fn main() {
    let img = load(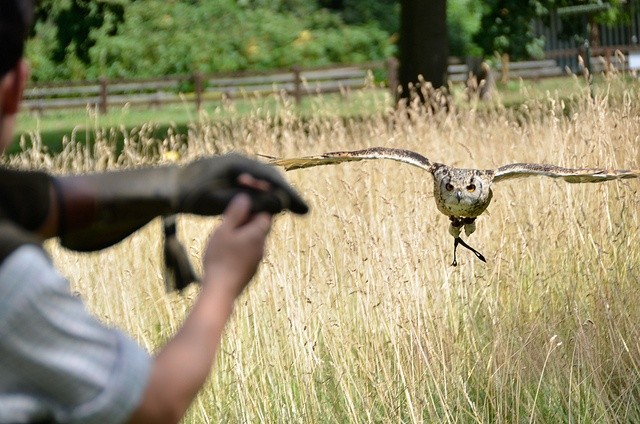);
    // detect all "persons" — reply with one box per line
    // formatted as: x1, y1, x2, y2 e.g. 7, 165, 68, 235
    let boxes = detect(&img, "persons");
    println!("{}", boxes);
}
0, 0, 309, 424
